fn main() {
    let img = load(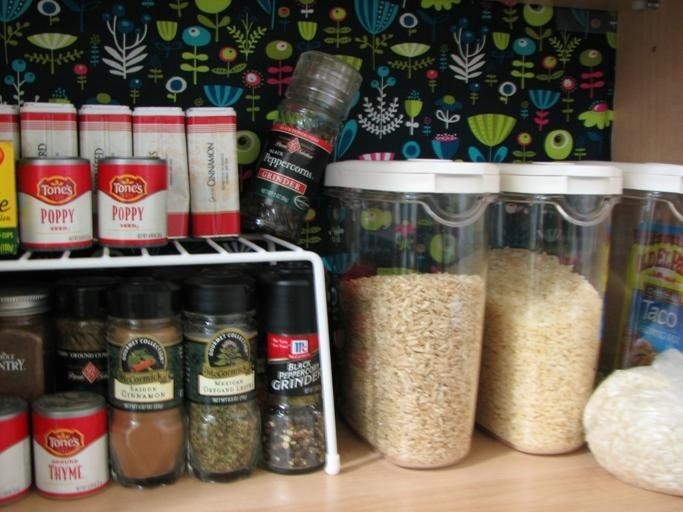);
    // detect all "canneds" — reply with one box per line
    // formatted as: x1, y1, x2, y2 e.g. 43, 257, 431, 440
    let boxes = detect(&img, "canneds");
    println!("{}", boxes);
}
1, 262, 326, 508
0, 102, 242, 252
